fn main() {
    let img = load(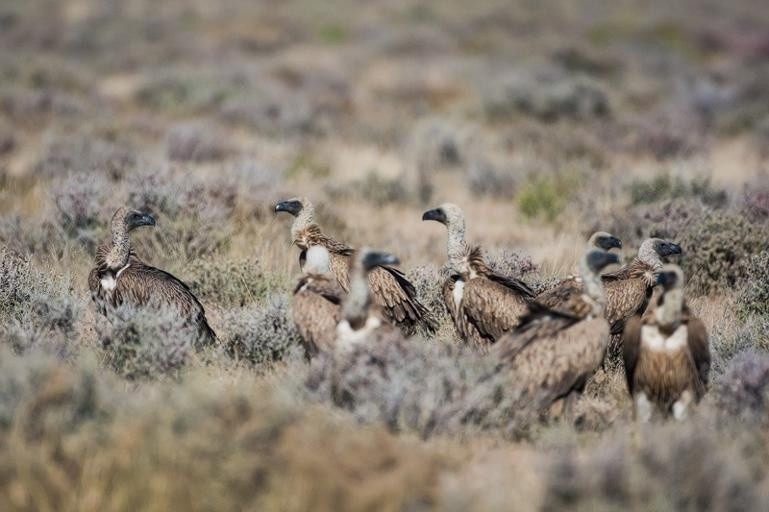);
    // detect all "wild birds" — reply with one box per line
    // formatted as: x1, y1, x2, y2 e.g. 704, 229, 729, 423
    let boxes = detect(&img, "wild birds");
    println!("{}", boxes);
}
85, 204, 222, 349
270, 195, 711, 426
